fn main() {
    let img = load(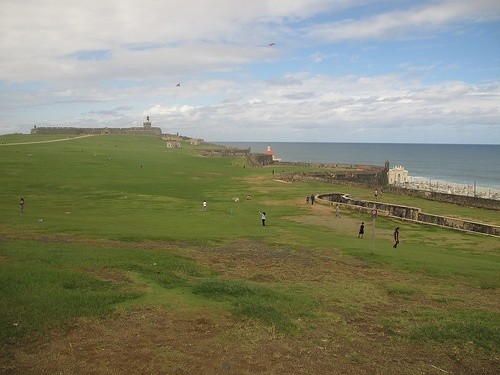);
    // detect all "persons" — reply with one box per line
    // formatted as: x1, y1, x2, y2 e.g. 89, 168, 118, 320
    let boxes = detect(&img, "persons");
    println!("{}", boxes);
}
306, 196, 309, 204
357, 222, 364, 239
393, 227, 399, 248
19, 198, 24, 215
201, 201, 208, 211
311, 194, 315, 205
335, 204, 340, 218
259, 211, 266, 226
